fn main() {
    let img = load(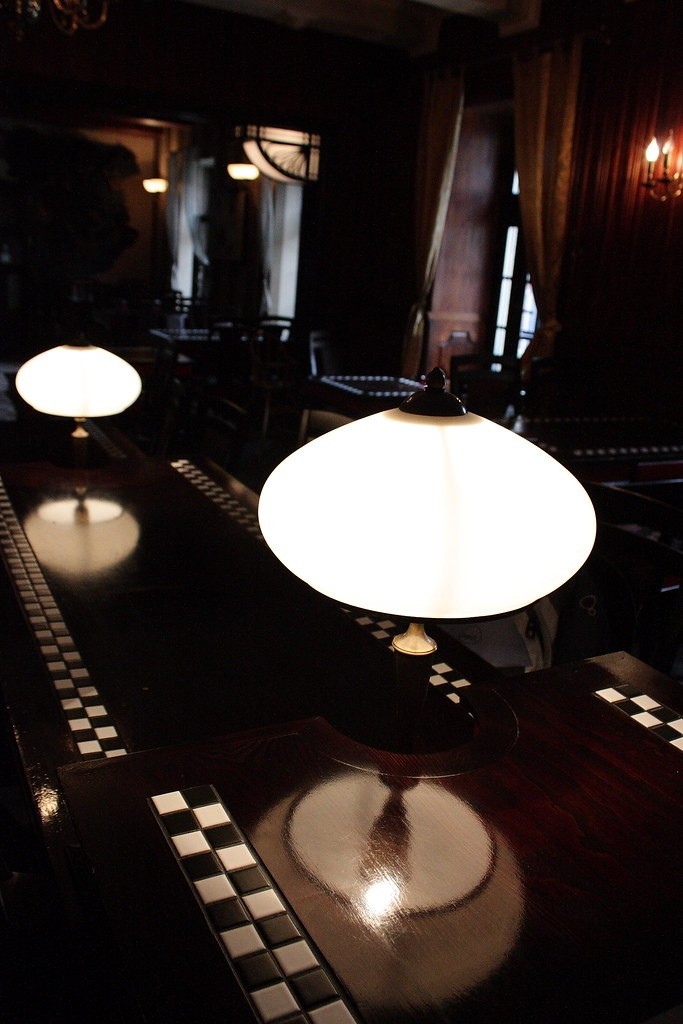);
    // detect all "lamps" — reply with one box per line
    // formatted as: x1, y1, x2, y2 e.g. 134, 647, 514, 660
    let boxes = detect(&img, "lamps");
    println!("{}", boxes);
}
236, 125, 322, 186
640, 129, 683, 205
16, 331, 142, 443
248, 772, 527, 1010
256, 357, 597, 785
24, 483, 142, 581
